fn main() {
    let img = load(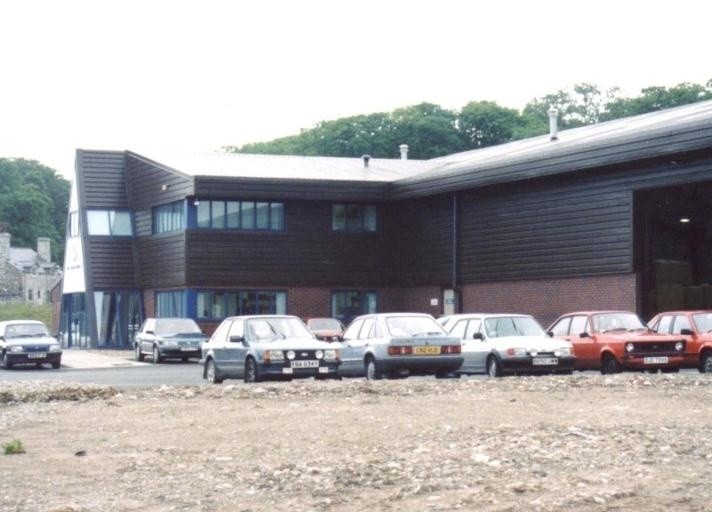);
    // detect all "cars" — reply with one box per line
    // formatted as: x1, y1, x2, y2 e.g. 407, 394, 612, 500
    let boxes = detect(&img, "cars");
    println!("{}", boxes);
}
441, 313, 574, 379
646, 310, 712, 374
133, 316, 209, 364
336, 312, 465, 380
306, 317, 346, 343
0, 319, 63, 369
545, 310, 687, 375
199, 314, 343, 383
